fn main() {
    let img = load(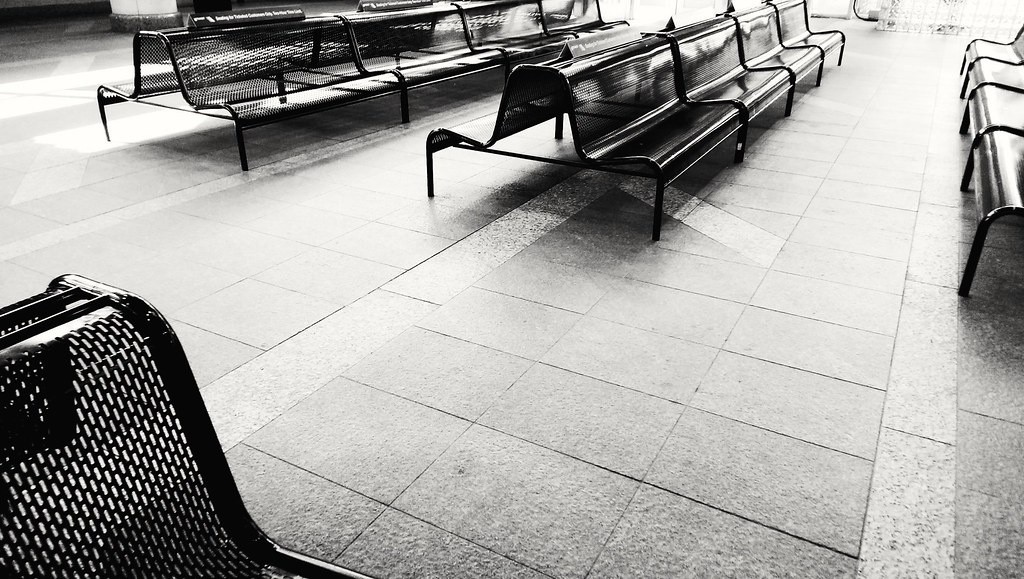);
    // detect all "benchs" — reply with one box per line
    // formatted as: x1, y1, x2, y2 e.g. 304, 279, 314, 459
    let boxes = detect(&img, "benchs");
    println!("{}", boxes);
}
96, 0, 848, 247
958, 24, 1024, 297
0, 273, 380, 579
